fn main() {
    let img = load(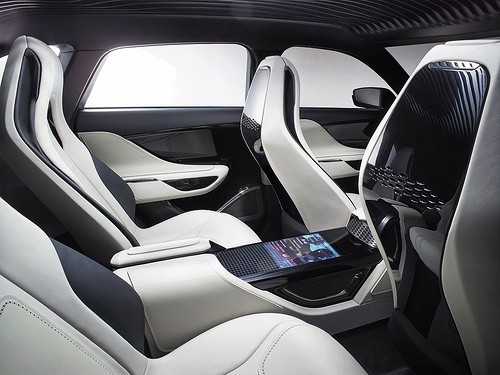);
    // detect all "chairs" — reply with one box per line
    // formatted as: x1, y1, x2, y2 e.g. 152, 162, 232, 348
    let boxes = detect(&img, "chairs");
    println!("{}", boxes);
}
0, 197, 370, 375
358, 39, 500, 375
0, 35, 262, 268
240, 55, 365, 237
